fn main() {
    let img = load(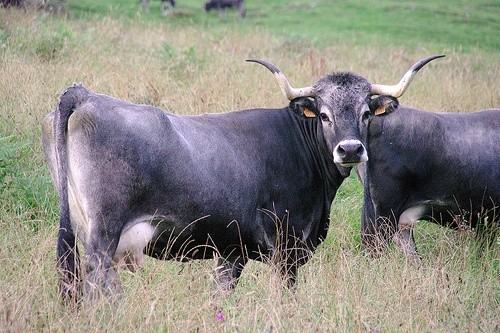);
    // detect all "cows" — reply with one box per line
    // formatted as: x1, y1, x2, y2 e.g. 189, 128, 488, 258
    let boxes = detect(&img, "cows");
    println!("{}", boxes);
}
355, 106, 500, 273
41, 54, 448, 308
